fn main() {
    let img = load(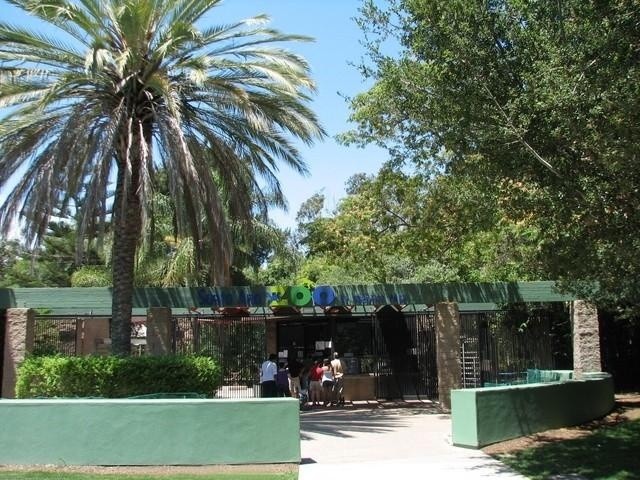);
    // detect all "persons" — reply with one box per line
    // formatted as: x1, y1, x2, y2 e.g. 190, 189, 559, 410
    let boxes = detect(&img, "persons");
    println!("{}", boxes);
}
330, 352, 347, 407
261, 353, 278, 398
284, 354, 304, 398
321, 358, 334, 407
309, 360, 323, 408
279, 362, 284, 372
